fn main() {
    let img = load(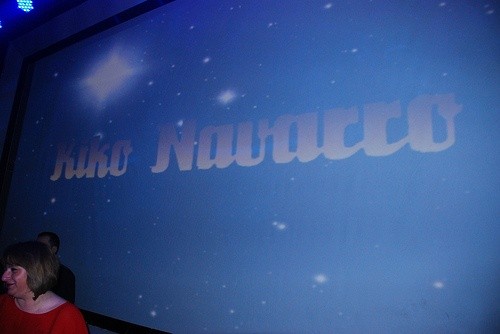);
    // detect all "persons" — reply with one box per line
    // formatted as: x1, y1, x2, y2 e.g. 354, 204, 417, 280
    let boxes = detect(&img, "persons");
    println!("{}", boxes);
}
0, 240, 89, 334
35, 232, 77, 305
